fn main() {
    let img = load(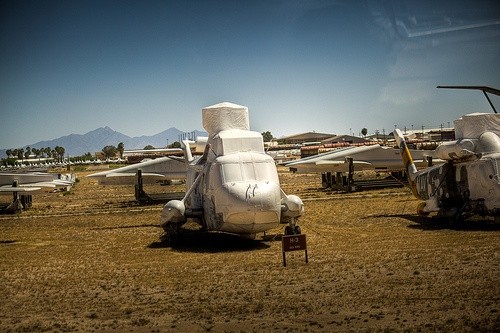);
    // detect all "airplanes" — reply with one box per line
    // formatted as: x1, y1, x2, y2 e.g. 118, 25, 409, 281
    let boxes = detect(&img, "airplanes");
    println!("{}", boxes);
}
5, 85, 500, 248
